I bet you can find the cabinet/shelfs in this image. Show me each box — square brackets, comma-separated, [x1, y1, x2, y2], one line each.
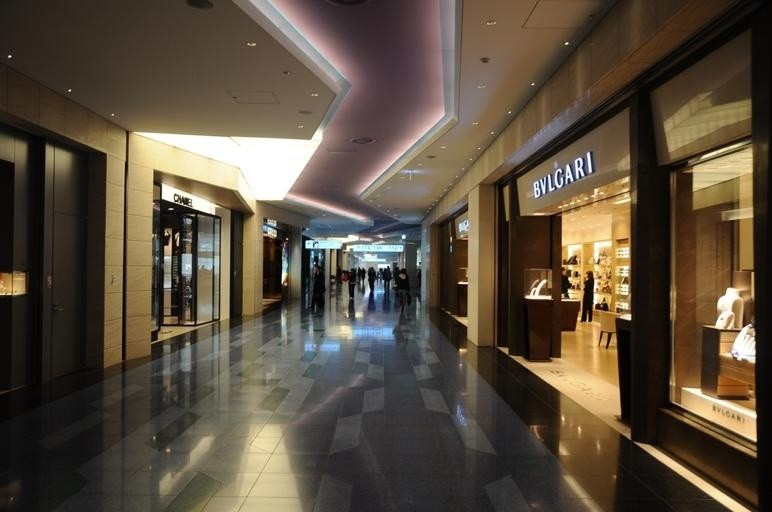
[457, 282, 468, 313]
[562, 243, 594, 317]
[700, 325, 754, 400]
[610, 238, 631, 315]
[561, 298, 581, 333]
[614, 316, 633, 429]
[582, 241, 611, 316]
[524, 294, 554, 363]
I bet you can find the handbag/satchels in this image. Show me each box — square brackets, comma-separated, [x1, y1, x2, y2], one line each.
[407, 294, 411, 306]
[596, 253, 611, 293]
[564, 255, 580, 289]
[595, 302, 609, 311]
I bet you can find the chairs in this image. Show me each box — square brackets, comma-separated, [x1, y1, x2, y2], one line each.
[597, 309, 621, 348]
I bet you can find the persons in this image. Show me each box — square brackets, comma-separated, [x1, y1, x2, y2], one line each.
[312, 256, 320, 285]
[580, 271, 595, 322]
[308, 264, 325, 312]
[561, 267, 574, 299]
[330, 262, 413, 314]
